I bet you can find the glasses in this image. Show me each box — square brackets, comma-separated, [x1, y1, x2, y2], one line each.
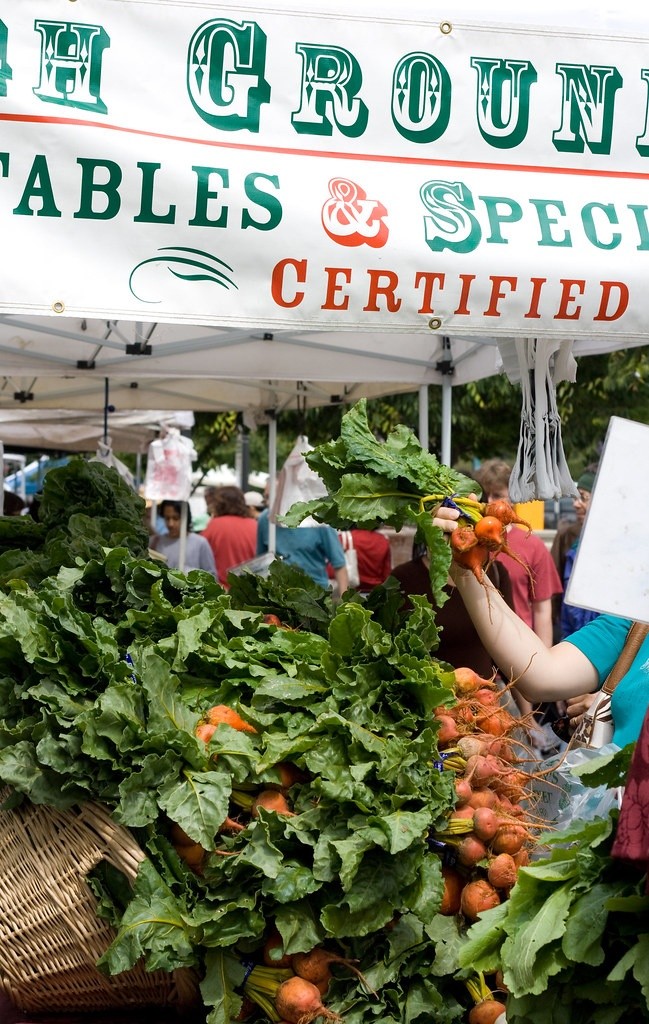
[571, 493, 592, 502]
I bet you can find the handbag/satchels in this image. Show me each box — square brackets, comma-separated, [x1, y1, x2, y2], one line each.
[569, 688, 615, 752]
[338, 530, 360, 587]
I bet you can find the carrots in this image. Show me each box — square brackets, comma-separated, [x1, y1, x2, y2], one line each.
[180, 665, 584, 1024]
[451, 500, 536, 625]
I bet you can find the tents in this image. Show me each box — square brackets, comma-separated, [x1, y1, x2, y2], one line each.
[1, 0, 648, 568]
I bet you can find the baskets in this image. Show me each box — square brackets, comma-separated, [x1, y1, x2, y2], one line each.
[0, 779, 204, 1017]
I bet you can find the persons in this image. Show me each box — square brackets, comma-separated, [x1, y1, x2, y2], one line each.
[198, 486, 267, 593]
[256, 474, 348, 599]
[362, 457, 649, 750]
[149, 499, 219, 578]
[325, 526, 393, 593]
